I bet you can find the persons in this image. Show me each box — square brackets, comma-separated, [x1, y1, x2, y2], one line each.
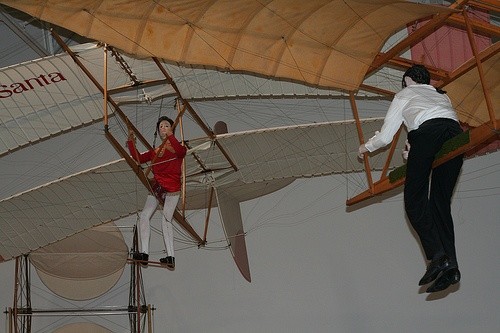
[357, 63, 465, 293]
[127, 116, 187, 269]
